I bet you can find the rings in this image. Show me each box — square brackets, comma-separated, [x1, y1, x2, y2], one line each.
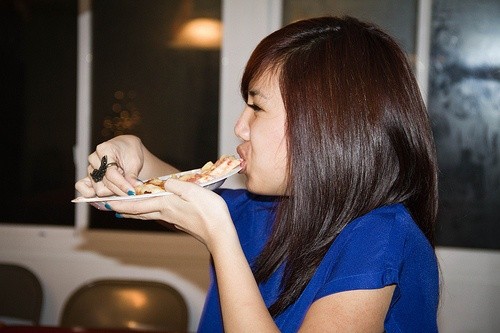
[90, 154, 119, 182]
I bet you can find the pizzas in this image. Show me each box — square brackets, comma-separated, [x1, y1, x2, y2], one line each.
[134, 155, 243, 195]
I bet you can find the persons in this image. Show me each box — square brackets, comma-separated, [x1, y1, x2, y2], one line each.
[74, 13, 441, 333]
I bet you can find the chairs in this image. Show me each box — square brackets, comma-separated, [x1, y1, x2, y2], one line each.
[58, 276, 190, 333]
[0, 261, 44, 326]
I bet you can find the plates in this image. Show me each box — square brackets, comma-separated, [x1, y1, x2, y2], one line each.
[71, 166, 243, 210]
